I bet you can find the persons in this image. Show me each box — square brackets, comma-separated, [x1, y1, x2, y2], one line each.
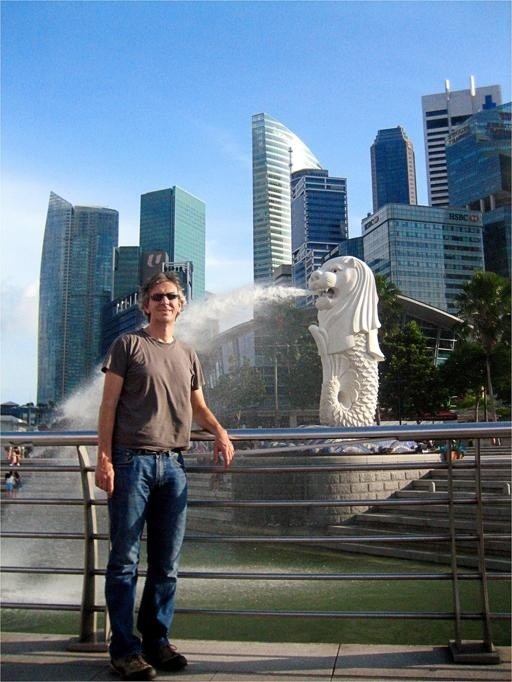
[6, 447, 22, 497]
[94, 273, 234, 680]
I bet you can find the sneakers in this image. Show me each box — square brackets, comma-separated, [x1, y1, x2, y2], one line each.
[143, 644, 188, 671]
[108, 654, 157, 681]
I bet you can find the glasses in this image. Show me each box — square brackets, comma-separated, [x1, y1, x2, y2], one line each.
[147, 292, 178, 302]
